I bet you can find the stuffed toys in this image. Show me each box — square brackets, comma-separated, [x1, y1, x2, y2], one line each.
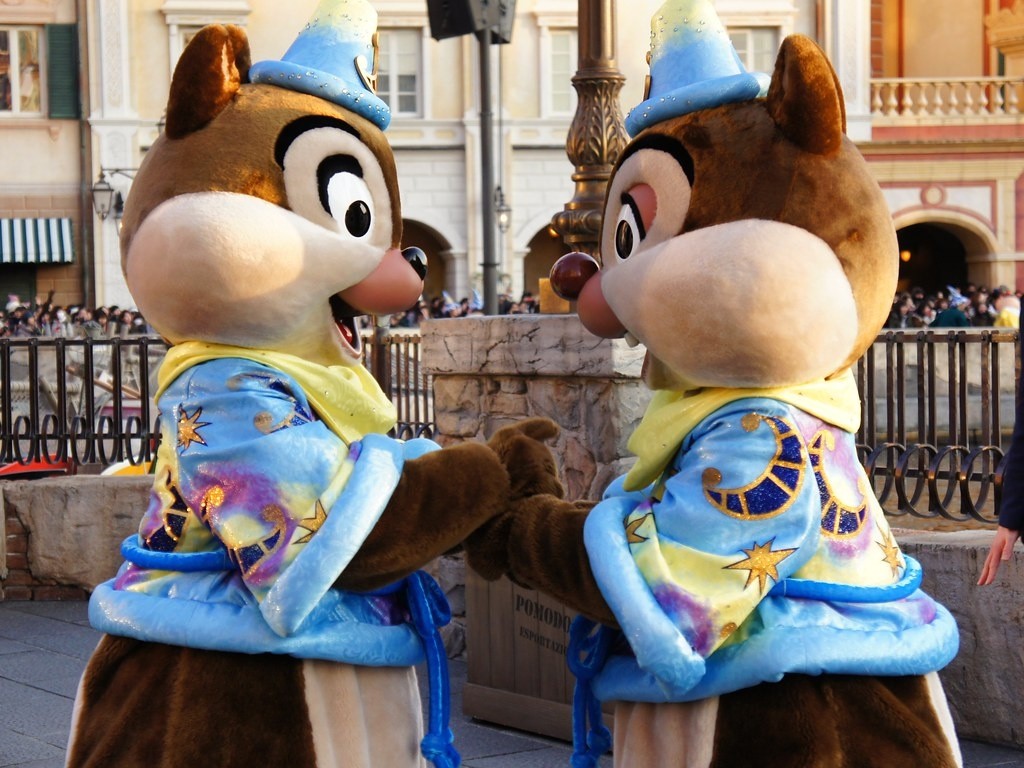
[64, 0, 564, 768]
[461, 0, 964, 768]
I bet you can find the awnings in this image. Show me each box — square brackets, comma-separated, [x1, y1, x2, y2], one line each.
[0, 217, 77, 264]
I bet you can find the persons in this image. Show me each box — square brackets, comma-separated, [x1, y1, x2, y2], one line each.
[977, 364, 1024, 586]
[0, 282, 156, 339]
[377, 289, 540, 330]
[883, 282, 1024, 329]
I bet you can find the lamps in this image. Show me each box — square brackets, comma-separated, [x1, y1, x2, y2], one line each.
[112, 192, 124, 235]
[90, 173, 115, 221]
